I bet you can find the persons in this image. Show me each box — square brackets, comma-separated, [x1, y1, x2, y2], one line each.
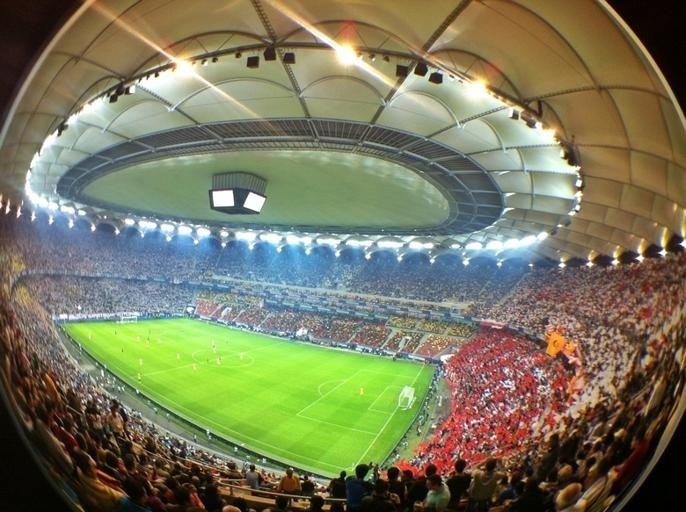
[2, 201, 686, 512]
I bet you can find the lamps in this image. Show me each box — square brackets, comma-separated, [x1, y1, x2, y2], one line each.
[107, 39, 444, 104]
